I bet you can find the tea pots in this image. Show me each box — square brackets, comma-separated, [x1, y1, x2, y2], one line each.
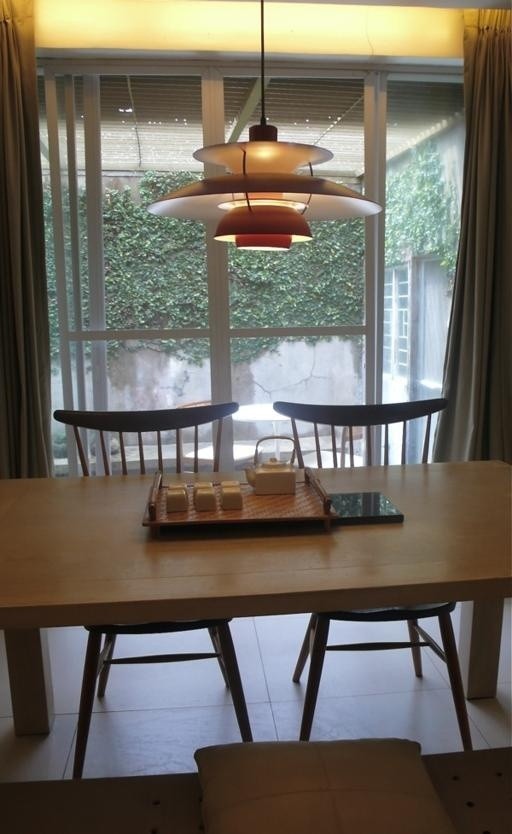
[245, 435, 301, 498]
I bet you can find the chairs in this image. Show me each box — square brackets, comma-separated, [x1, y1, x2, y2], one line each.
[271, 395, 474, 754]
[53, 402, 253, 781]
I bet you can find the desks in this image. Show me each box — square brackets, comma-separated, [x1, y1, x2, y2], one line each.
[0, 458, 512, 739]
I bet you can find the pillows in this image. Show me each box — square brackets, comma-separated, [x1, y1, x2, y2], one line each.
[193, 738, 457, 833]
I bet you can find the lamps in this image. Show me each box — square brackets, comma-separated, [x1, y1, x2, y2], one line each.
[148, 1, 384, 252]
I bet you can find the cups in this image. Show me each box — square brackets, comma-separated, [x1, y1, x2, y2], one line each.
[164, 480, 245, 513]
